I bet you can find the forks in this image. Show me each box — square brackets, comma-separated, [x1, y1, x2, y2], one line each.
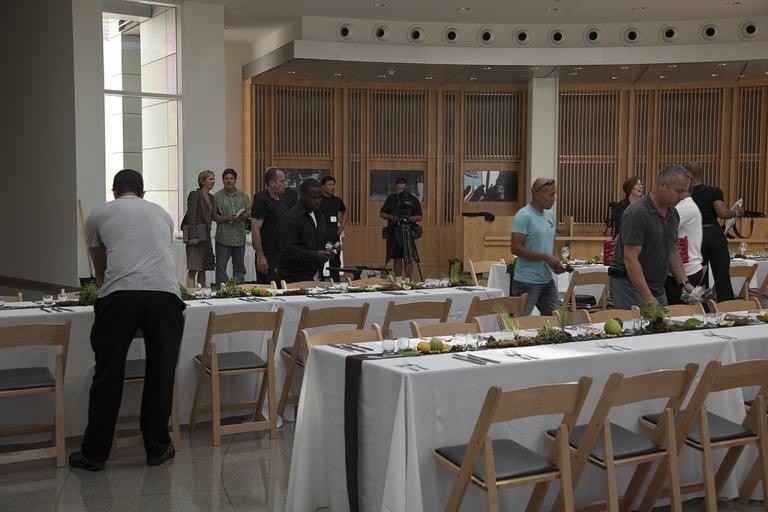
[503, 349, 539, 359]
[511, 350, 531, 360]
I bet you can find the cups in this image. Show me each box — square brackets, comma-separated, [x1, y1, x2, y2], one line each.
[382, 339, 394, 357]
[560, 247, 570, 259]
[396, 337, 409, 356]
[58, 294, 67, 302]
[739, 242, 748, 258]
[467, 333, 478, 351]
[43, 295, 53, 304]
[202, 288, 211, 298]
[194, 288, 202, 298]
[455, 333, 467, 351]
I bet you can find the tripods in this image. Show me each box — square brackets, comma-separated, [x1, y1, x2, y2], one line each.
[383, 223, 424, 282]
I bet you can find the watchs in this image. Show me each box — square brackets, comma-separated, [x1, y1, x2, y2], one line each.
[735, 210, 738, 217]
[679, 279, 690, 287]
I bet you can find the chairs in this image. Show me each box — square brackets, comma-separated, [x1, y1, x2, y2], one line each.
[705, 297, 762, 312]
[750, 274, 768, 302]
[546, 361, 702, 512]
[106, 311, 187, 451]
[640, 358, 768, 511]
[300, 323, 383, 350]
[579, 305, 641, 325]
[465, 291, 531, 324]
[382, 297, 452, 336]
[0, 317, 74, 469]
[497, 312, 560, 333]
[564, 270, 610, 322]
[653, 301, 705, 314]
[714, 263, 758, 298]
[189, 307, 285, 447]
[409, 319, 483, 337]
[432, 374, 593, 512]
[278, 302, 371, 433]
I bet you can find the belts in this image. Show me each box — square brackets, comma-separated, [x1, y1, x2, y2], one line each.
[702, 223, 715, 227]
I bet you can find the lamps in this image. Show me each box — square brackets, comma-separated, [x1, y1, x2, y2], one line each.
[543, 6, 559, 13]
[457, 5, 471, 12]
[728, 0, 742, 7]
[372, 1, 386, 9]
[630, 6, 646, 12]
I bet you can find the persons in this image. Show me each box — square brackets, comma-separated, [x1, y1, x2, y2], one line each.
[380, 178, 423, 282]
[683, 161, 745, 302]
[179, 170, 217, 287]
[677, 178, 703, 306]
[607, 164, 694, 309]
[250, 166, 288, 282]
[212, 167, 252, 284]
[69, 168, 187, 474]
[613, 175, 643, 221]
[320, 177, 349, 281]
[277, 177, 336, 282]
[511, 177, 566, 317]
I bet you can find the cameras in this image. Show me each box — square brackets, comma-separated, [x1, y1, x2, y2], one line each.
[396, 189, 412, 223]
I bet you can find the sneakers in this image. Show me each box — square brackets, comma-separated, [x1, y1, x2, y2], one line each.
[147, 441, 175, 466]
[69, 451, 103, 472]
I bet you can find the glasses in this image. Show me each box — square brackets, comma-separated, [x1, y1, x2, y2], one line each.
[536, 178, 555, 191]
